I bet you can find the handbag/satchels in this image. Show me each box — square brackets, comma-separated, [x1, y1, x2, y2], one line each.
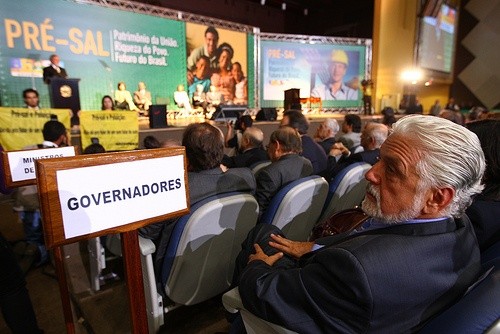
[315, 207, 368, 238]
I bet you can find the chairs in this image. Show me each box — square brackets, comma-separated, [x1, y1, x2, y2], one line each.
[165, 128, 499, 334]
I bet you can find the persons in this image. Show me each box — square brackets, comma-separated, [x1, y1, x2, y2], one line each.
[43, 55, 66, 84]
[133, 82, 152, 115]
[192, 84, 221, 114]
[83, 98, 485, 303]
[101, 95, 113, 110]
[465, 119, 500, 258]
[188, 26, 248, 106]
[223, 114, 487, 334]
[22, 89, 40, 109]
[174, 84, 191, 109]
[114, 81, 144, 113]
[311, 49, 358, 100]
[11, 120, 67, 211]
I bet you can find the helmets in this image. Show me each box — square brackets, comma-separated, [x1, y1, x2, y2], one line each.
[328, 48, 348, 68]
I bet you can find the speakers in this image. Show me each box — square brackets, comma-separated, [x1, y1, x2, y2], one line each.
[255, 107, 278, 121]
[150, 104, 168, 128]
